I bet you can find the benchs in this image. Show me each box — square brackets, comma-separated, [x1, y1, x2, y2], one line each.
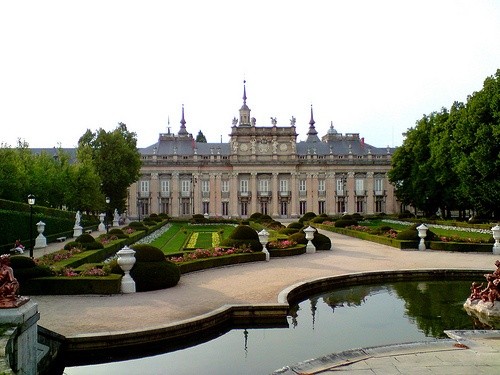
[85, 230, 92, 234]
[56, 236, 67, 242]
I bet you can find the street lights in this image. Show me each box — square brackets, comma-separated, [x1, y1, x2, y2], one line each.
[191, 175, 198, 214]
[341, 174, 347, 213]
[105, 197, 110, 233]
[27, 195, 37, 259]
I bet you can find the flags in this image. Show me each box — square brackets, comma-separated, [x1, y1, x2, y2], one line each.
[191, 138, 198, 150]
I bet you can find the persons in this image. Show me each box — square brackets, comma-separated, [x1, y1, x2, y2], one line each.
[14, 238, 25, 250]
[0, 254, 19, 300]
[470, 260, 500, 303]
